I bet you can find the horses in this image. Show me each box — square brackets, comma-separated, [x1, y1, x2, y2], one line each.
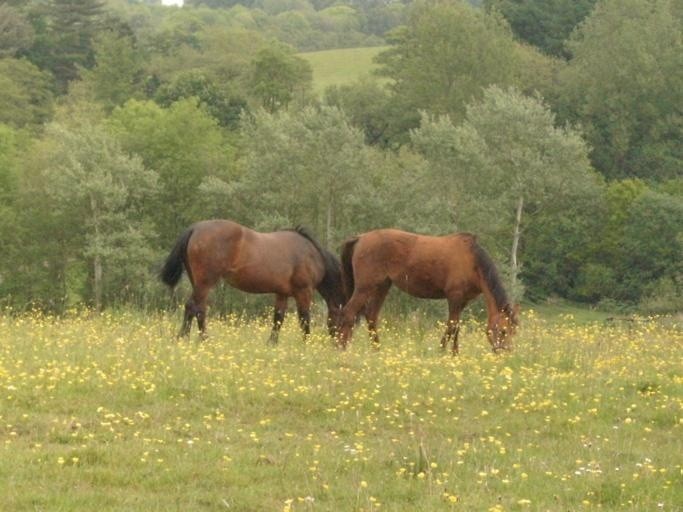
[337, 228, 519, 354]
[158, 219, 354, 348]
